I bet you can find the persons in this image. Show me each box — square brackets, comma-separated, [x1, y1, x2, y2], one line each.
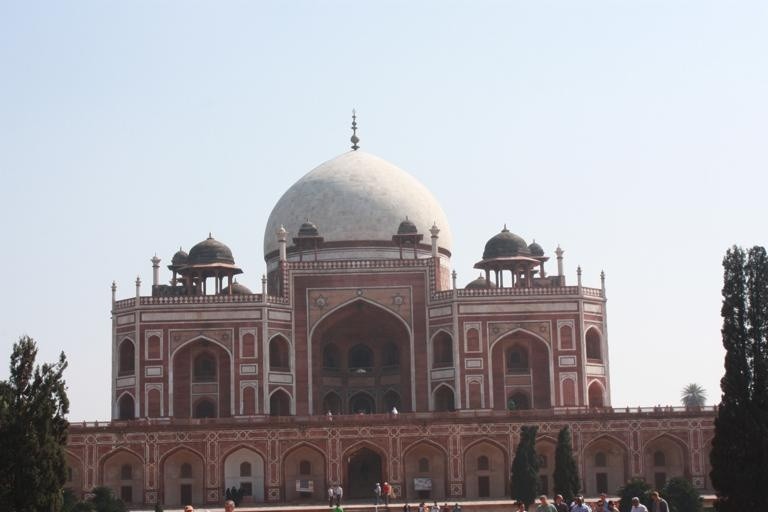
[184, 505, 193, 512]
[335, 484, 343, 504]
[383, 481, 390, 505]
[326, 409, 332, 421]
[328, 484, 334, 507]
[553, 494, 569, 512]
[630, 496, 648, 512]
[388, 482, 396, 503]
[515, 502, 528, 512]
[536, 494, 559, 512]
[225, 499, 235, 512]
[418, 500, 461, 512]
[570, 492, 620, 511]
[651, 491, 670, 511]
[404, 502, 411, 512]
[374, 482, 382, 505]
